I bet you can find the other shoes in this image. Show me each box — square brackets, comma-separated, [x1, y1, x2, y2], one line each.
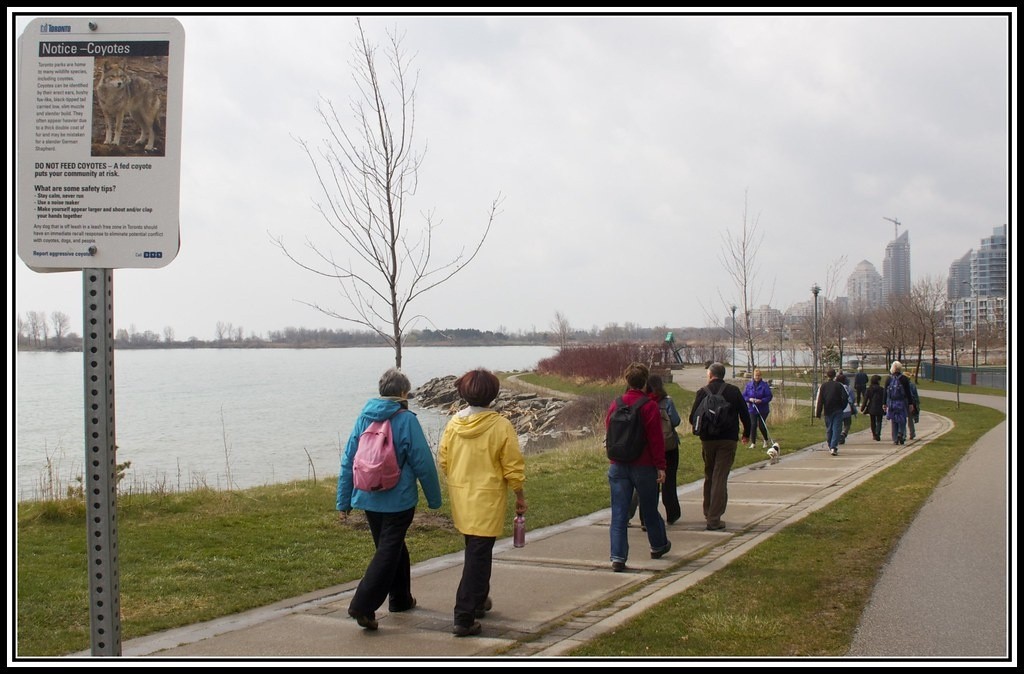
[839, 434, 844, 444]
[874, 434, 880, 441]
[708, 521, 725, 530]
[412, 597, 416, 608]
[348, 607, 378, 629]
[898, 436, 904, 444]
[831, 448, 838, 455]
[641, 521, 647, 530]
[910, 434, 915, 439]
[894, 442, 898, 445]
[762, 440, 767, 448]
[749, 443, 755, 448]
[627, 522, 633, 527]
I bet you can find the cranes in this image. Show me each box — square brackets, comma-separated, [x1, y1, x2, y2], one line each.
[882, 216, 901, 240]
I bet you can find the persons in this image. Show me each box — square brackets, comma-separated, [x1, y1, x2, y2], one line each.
[688, 361, 752, 533]
[599, 363, 671, 571]
[853, 367, 868, 408]
[638, 373, 680, 533]
[900, 371, 921, 441]
[742, 369, 774, 449]
[335, 368, 444, 630]
[815, 369, 848, 457]
[834, 371, 856, 446]
[860, 374, 886, 442]
[882, 360, 913, 445]
[437, 371, 527, 637]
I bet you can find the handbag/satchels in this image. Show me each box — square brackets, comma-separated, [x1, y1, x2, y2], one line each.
[863, 403, 870, 414]
[851, 404, 858, 415]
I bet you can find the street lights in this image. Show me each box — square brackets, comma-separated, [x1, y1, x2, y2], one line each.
[731, 304, 737, 378]
[811, 282, 822, 401]
[963, 281, 979, 368]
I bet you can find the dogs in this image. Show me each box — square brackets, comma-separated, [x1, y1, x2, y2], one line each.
[766, 442, 780, 461]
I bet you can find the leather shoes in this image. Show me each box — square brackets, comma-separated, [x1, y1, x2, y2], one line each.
[475, 597, 492, 619]
[651, 540, 671, 559]
[451, 621, 481, 635]
[612, 562, 625, 572]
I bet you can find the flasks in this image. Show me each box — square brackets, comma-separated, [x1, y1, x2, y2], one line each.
[514, 514, 525, 548]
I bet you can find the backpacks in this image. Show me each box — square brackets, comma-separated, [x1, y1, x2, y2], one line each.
[659, 398, 678, 453]
[604, 394, 652, 463]
[351, 408, 409, 491]
[691, 382, 732, 437]
[888, 374, 906, 400]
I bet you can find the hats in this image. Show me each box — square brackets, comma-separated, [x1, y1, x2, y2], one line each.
[827, 369, 836, 378]
